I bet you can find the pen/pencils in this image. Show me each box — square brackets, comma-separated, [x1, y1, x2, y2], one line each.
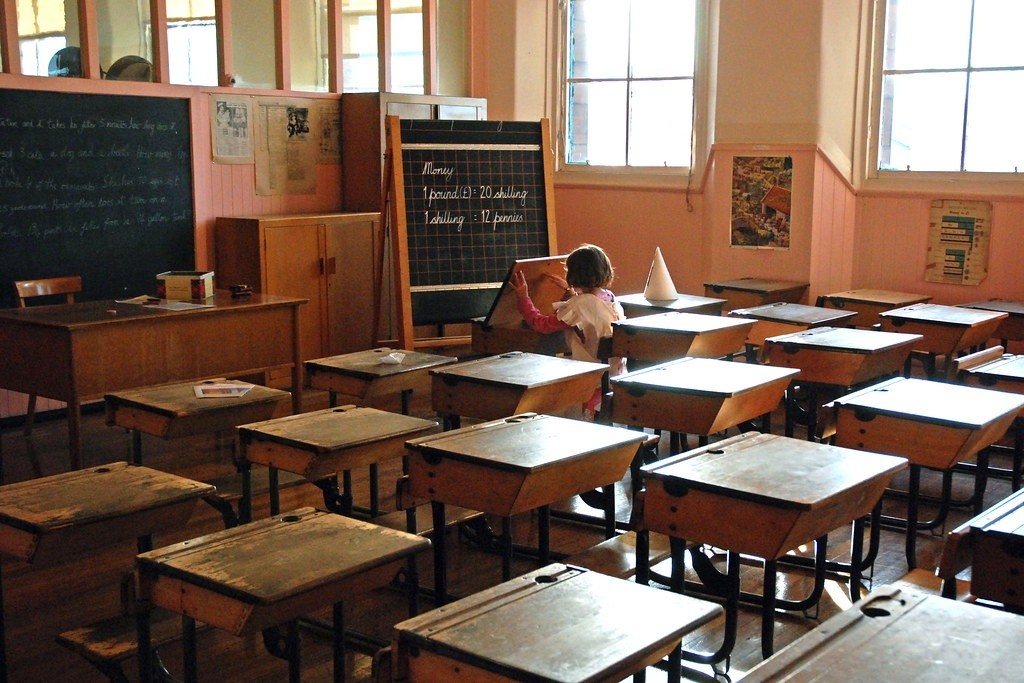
[142, 298, 161, 305]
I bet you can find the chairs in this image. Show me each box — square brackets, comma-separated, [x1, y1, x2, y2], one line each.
[901, 488, 1024, 602]
[587, 393, 660, 463]
[946, 344, 1004, 384]
[55, 531, 217, 683]
[15, 275, 86, 436]
[592, 337, 612, 414]
[363, 475, 488, 588]
[563, 431, 758, 578]
[814, 376, 906, 446]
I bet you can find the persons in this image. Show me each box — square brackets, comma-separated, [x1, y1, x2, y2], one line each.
[507, 245, 629, 423]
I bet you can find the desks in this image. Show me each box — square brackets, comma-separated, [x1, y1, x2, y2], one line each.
[0, 255, 1024, 683]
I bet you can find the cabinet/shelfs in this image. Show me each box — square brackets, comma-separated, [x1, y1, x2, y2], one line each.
[340, 92, 488, 346]
[216, 211, 390, 390]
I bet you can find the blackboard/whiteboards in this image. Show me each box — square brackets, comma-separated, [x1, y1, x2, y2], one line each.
[0, 73, 213, 311]
[387, 114, 559, 294]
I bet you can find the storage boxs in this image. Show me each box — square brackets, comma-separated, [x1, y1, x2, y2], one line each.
[155, 270, 216, 302]
